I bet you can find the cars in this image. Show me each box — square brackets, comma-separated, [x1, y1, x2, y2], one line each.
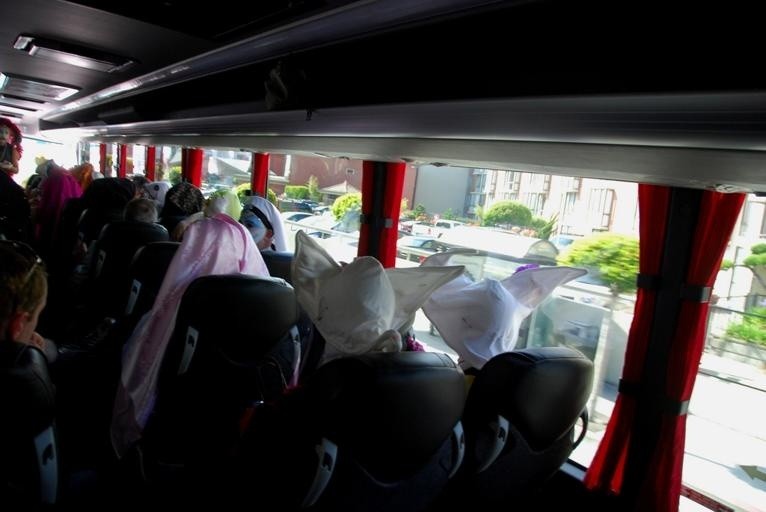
[201, 165, 580, 270]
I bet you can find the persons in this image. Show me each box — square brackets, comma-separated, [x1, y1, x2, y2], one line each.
[0, 117, 546, 512]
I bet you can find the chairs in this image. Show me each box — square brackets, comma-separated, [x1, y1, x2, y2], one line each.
[0, 177, 594, 512]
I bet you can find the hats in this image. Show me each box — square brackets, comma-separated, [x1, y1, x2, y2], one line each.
[417, 246, 587, 371]
[245, 195, 286, 252]
[289, 229, 465, 357]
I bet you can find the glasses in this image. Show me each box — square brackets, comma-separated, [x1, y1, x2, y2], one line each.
[1, 231, 42, 286]
